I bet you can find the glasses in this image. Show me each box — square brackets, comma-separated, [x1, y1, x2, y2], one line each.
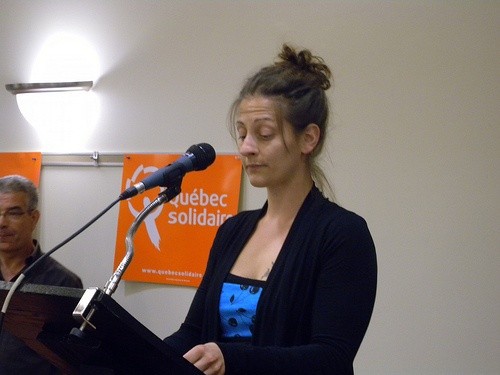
[0, 208, 35, 219]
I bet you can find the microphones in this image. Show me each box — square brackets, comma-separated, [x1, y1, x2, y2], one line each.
[119, 143, 216, 200]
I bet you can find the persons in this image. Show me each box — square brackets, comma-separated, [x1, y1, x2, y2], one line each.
[164, 44, 378, 375]
[0, 175, 83, 375]
